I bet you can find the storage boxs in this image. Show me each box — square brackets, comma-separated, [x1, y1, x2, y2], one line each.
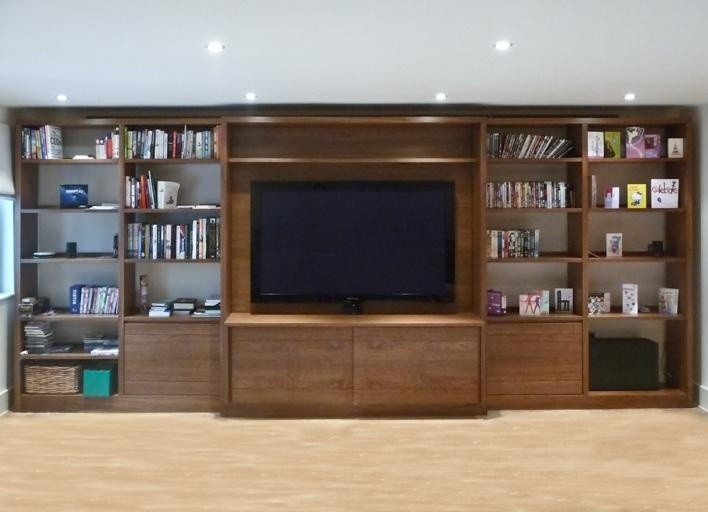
[83, 364, 117, 398]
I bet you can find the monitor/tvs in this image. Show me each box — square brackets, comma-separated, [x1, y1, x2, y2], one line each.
[248, 178, 456, 316]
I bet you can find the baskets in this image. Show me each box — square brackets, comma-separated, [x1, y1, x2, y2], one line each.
[22, 363, 83, 393]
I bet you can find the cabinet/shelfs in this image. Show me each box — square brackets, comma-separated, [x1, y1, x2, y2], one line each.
[224, 313, 486, 420]
[481, 118, 693, 410]
[15, 118, 227, 414]
[222, 117, 484, 325]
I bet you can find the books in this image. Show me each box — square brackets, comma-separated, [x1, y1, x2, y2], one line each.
[589, 293, 611, 313]
[95, 128, 119, 159]
[626, 127, 661, 158]
[519, 290, 550, 317]
[590, 175, 597, 207]
[605, 233, 623, 257]
[555, 288, 574, 314]
[601, 186, 620, 210]
[80, 327, 119, 356]
[589, 132, 603, 157]
[172, 296, 198, 317]
[651, 179, 679, 208]
[149, 303, 173, 318]
[22, 322, 74, 358]
[124, 124, 214, 159]
[487, 229, 539, 257]
[668, 138, 684, 158]
[605, 131, 621, 158]
[486, 180, 577, 209]
[622, 283, 639, 315]
[126, 172, 180, 208]
[657, 288, 679, 314]
[487, 290, 508, 316]
[204, 299, 221, 315]
[68, 285, 118, 314]
[626, 184, 647, 208]
[21, 126, 63, 159]
[488, 133, 575, 159]
[127, 217, 220, 259]
